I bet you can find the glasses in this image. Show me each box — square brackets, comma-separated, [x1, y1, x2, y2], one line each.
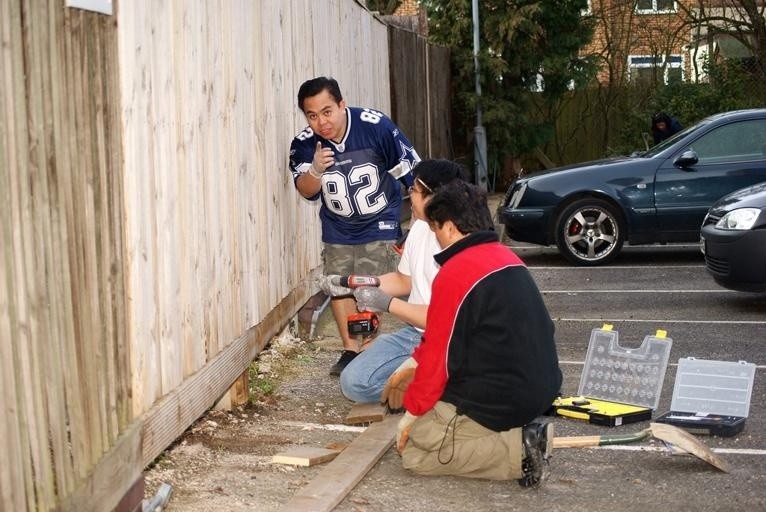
[408, 186, 422, 194]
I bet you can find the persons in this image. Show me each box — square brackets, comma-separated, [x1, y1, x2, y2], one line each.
[288, 78, 423, 378]
[378, 179, 565, 490]
[652, 113, 684, 145]
[316, 156, 471, 402]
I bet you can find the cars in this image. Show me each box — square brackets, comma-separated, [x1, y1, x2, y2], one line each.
[699, 179, 765, 294]
[497, 108, 765, 268]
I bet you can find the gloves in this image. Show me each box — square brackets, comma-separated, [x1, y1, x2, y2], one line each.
[353, 286, 394, 313]
[319, 275, 354, 297]
[309, 141, 335, 179]
[397, 411, 418, 456]
[381, 357, 419, 409]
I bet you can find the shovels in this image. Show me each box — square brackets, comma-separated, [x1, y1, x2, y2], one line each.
[553, 422, 730, 474]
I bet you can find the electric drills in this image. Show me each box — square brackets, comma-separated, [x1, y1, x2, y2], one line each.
[331, 275, 380, 335]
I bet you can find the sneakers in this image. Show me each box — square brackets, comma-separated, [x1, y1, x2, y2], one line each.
[330, 350, 357, 376]
[519, 422, 554, 488]
